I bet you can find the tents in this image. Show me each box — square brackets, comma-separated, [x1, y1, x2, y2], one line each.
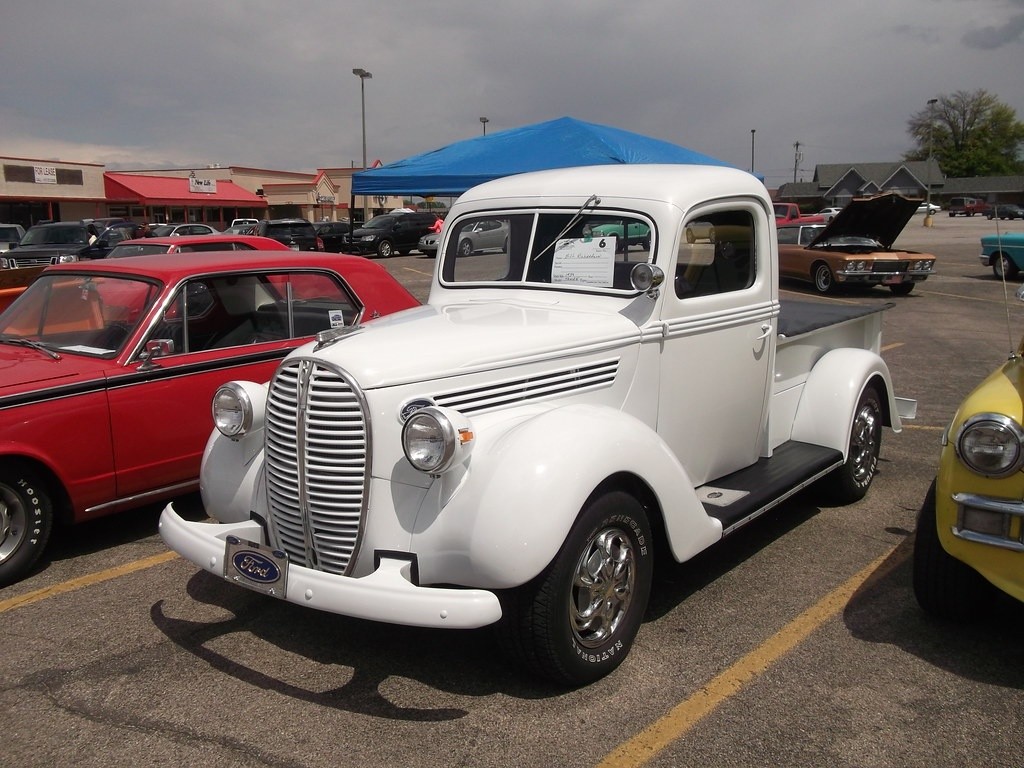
[349, 116, 764, 256]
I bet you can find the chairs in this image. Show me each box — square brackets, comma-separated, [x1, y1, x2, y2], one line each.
[159, 322, 186, 348]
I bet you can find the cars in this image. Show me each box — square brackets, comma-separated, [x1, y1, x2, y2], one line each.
[915, 197, 1024, 281]
[93, 235, 343, 322]
[148, 219, 259, 238]
[912, 336, 1024, 633]
[777, 192, 937, 295]
[417, 220, 509, 258]
[686, 222, 716, 244]
[583, 220, 651, 255]
[0, 224, 26, 254]
[0, 250, 424, 585]
[342, 212, 439, 258]
[312, 222, 357, 253]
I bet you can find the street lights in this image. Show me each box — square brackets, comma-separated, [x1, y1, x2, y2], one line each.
[927, 99, 939, 218]
[352, 69, 373, 224]
[480, 117, 489, 135]
[750, 129, 755, 172]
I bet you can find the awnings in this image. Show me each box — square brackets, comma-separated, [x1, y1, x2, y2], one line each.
[103, 173, 268, 209]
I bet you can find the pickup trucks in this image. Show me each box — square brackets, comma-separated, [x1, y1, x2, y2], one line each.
[158, 164, 920, 688]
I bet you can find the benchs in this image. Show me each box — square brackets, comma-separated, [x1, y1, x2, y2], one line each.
[615, 261, 747, 295]
[206, 311, 337, 349]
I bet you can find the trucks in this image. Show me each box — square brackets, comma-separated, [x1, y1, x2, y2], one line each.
[773, 203, 825, 227]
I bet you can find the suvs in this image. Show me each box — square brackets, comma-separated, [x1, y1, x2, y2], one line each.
[0, 217, 158, 270]
[246, 218, 319, 252]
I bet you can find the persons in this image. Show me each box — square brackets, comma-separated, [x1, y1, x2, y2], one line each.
[427, 215, 444, 233]
[88, 224, 98, 246]
[136, 222, 151, 239]
